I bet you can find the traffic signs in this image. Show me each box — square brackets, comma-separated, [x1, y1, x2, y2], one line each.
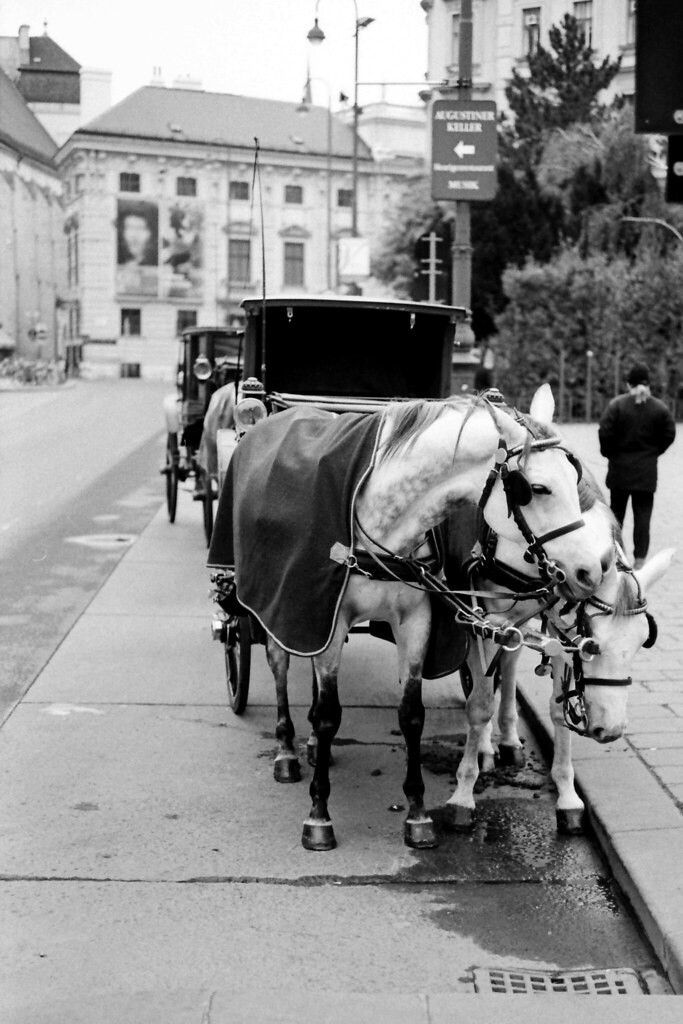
[430, 100, 501, 202]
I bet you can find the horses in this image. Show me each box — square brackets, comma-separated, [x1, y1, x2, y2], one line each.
[438, 450, 650, 836]
[231, 381, 604, 852]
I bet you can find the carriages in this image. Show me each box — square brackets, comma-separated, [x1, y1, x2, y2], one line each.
[201, 298, 682, 853]
[157, 325, 248, 548]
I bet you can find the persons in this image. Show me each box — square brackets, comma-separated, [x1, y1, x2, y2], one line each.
[597, 362, 676, 573]
[118, 212, 157, 266]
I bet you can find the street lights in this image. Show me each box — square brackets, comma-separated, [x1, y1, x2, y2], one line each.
[296, 73, 333, 291]
[307, 0, 378, 295]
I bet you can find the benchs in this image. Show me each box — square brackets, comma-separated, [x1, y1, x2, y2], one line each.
[239, 296, 468, 416]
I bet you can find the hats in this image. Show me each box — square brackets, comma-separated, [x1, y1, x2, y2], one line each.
[627, 361, 650, 384]
